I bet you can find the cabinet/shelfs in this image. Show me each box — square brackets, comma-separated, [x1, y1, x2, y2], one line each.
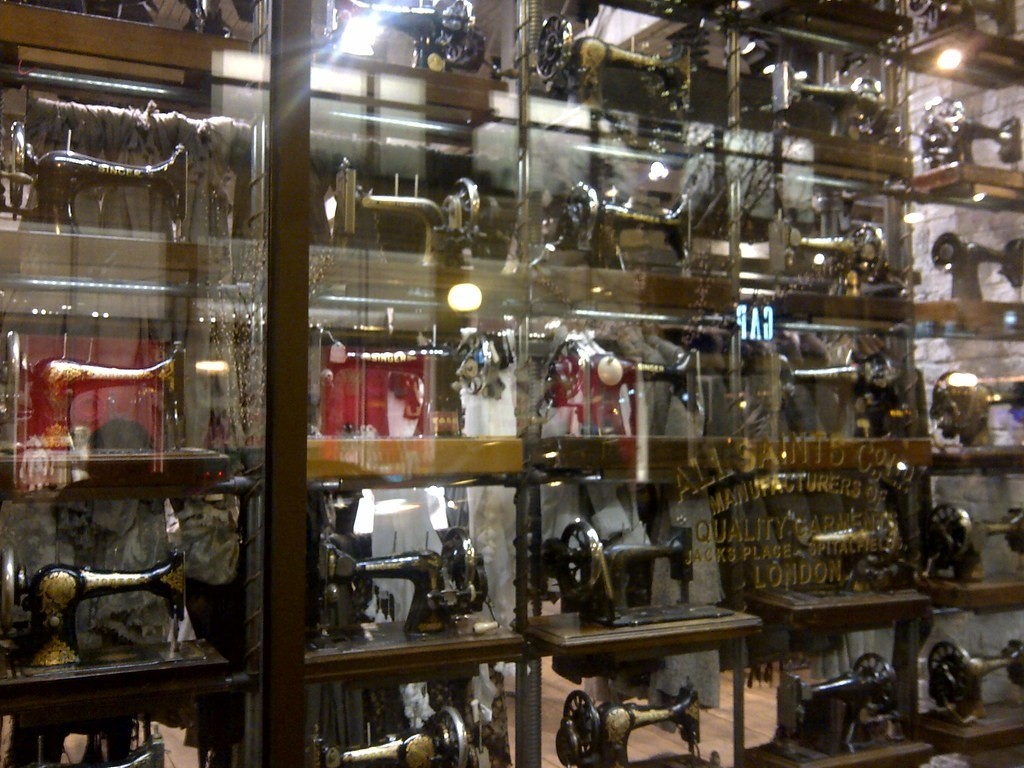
[0, 0, 1024, 768]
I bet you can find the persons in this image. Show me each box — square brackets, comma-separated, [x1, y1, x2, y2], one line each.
[29, 419, 187, 768]
